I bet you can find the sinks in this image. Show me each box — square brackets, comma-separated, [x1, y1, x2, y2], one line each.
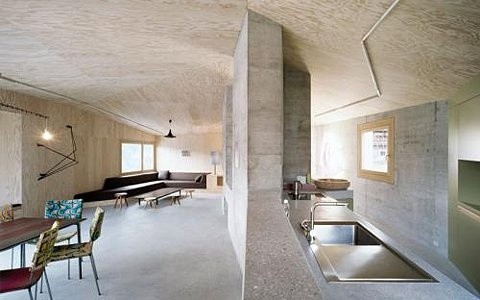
[301, 220, 383, 247]
[287, 190, 326, 201]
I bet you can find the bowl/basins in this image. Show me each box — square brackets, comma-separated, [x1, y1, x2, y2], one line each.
[314, 178, 350, 190]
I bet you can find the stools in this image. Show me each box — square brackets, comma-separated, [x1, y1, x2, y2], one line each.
[114, 193, 128, 209]
[167, 195, 181, 206]
[184, 189, 194, 198]
[144, 197, 157, 208]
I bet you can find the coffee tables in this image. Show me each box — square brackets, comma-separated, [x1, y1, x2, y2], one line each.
[134, 188, 183, 205]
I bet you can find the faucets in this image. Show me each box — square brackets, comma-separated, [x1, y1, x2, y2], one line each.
[293, 180, 302, 195]
[308, 202, 350, 231]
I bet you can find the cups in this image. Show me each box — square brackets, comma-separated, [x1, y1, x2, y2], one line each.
[293, 181, 302, 192]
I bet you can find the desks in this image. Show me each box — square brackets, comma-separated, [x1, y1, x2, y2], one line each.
[0, 217, 88, 279]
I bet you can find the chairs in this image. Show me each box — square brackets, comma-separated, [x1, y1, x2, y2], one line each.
[0, 199, 104, 300]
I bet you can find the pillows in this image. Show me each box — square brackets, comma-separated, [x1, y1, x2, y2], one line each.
[158, 170, 169, 180]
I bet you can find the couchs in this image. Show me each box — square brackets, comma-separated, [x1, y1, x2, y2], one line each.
[74, 172, 212, 203]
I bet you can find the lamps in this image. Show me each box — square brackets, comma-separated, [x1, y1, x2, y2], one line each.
[164, 129, 176, 139]
